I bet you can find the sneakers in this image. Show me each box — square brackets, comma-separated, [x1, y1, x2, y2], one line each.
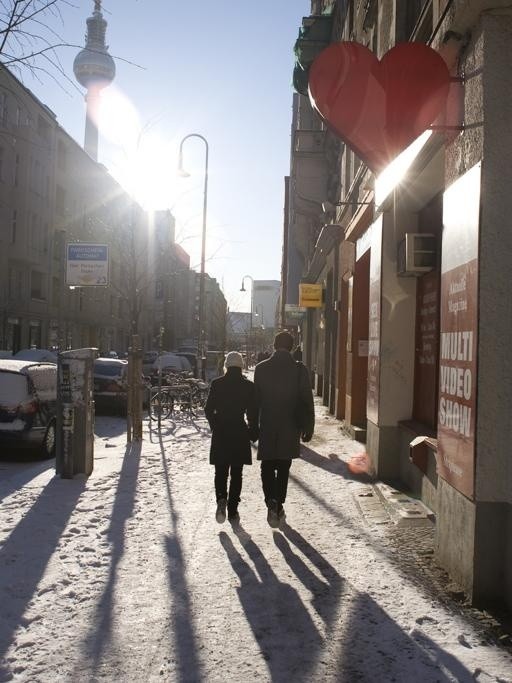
[228, 511, 238, 520]
[267, 499, 286, 528]
[216, 500, 226, 523]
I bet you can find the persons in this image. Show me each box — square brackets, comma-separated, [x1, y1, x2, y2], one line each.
[203, 349, 261, 524]
[253, 330, 316, 528]
[292, 344, 303, 362]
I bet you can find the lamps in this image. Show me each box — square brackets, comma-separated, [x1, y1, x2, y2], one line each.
[322, 199, 375, 213]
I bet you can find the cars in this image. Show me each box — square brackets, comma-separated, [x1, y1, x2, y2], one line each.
[0, 348, 200, 459]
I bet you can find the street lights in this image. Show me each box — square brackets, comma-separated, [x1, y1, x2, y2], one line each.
[174, 133, 208, 381]
[240, 274, 263, 370]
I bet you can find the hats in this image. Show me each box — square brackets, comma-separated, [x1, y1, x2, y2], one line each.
[226, 352, 242, 368]
[274, 333, 294, 351]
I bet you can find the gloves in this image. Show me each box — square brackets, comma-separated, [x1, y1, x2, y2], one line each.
[249, 425, 259, 443]
[301, 432, 312, 442]
